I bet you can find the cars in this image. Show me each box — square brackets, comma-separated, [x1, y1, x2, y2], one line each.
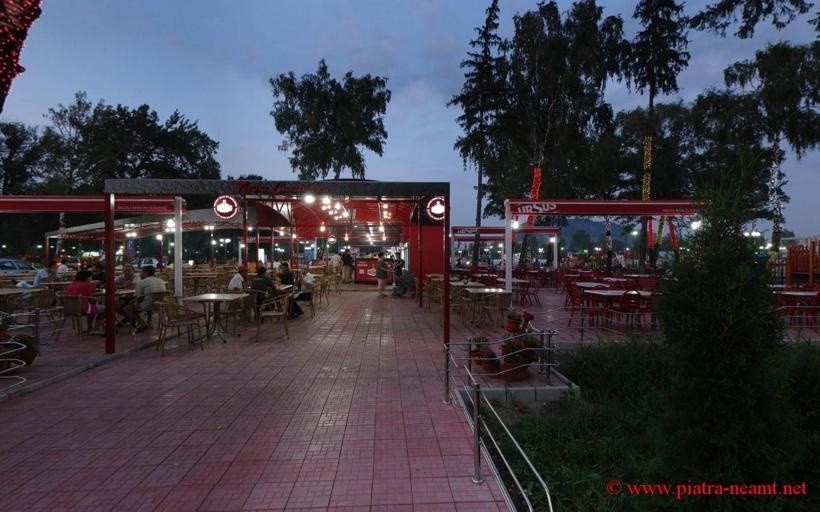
[0, 258, 39, 276]
[115, 257, 159, 269]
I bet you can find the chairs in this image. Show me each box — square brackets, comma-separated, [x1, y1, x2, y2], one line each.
[1, 224, 819, 379]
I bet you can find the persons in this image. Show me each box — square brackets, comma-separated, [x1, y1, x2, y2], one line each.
[391, 252, 405, 290]
[228, 257, 315, 320]
[533, 259, 540, 269]
[376, 252, 390, 298]
[33, 258, 107, 335]
[454, 259, 461, 268]
[322, 249, 378, 284]
[122, 265, 166, 334]
[115, 263, 141, 328]
